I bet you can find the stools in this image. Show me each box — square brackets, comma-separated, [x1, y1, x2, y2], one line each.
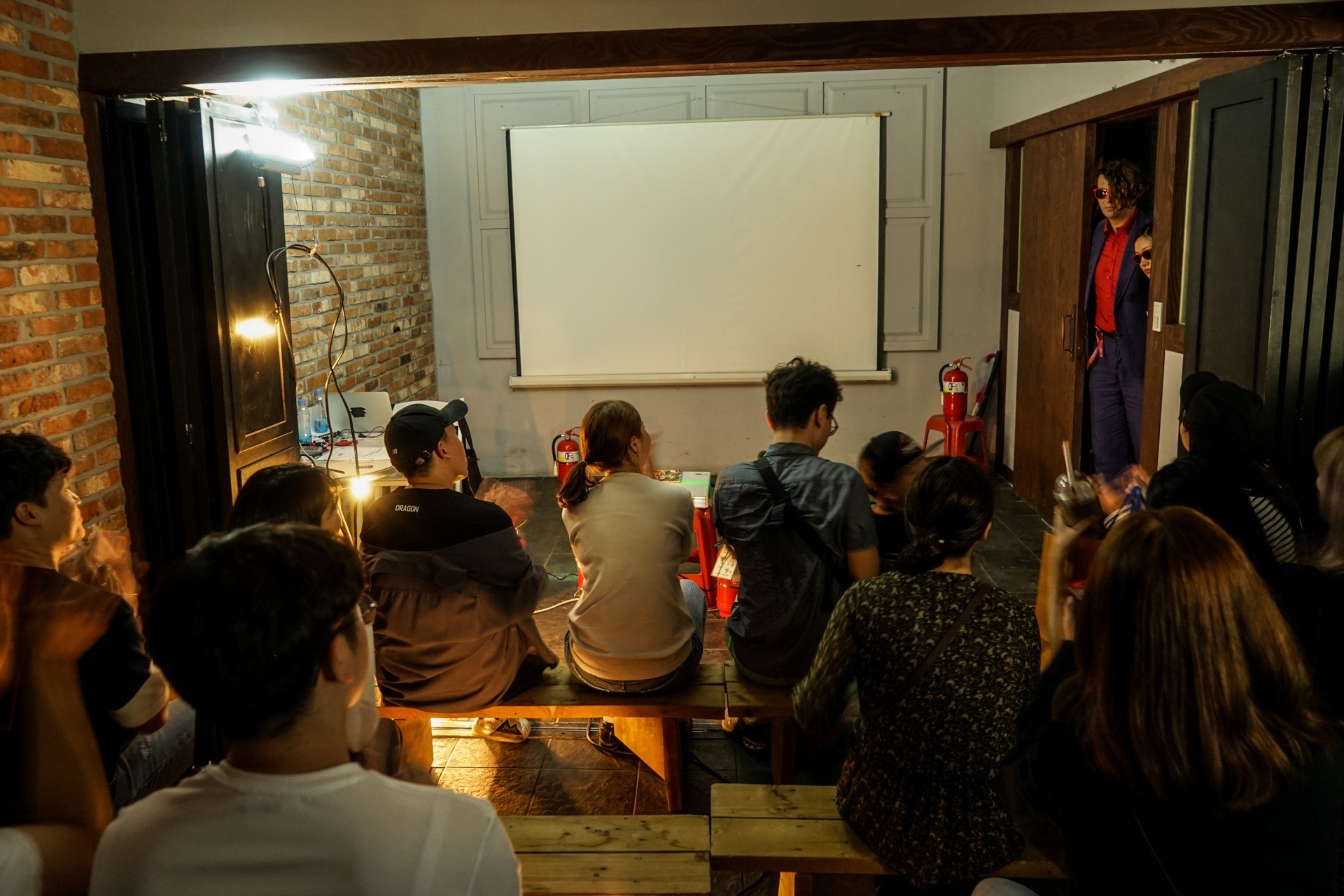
[920, 414, 988, 478]
[656, 497, 728, 617]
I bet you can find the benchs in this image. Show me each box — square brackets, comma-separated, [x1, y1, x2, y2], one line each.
[497, 808, 709, 896]
[710, 641, 836, 781]
[708, 778, 888, 896]
[363, 653, 725, 810]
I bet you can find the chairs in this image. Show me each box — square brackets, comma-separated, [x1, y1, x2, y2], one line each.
[374, 398, 462, 510]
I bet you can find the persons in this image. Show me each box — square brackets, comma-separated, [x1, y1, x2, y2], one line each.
[360, 400, 560, 714]
[859, 429, 944, 578]
[1083, 158, 1152, 482]
[793, 454, 1045, 890]
[1, 433, 527, 896]
[711, 356, 878, 691]
[1043, 373, 1344, 896]
[552, 400, 707, 698]
[1132, 222, 1153, 279]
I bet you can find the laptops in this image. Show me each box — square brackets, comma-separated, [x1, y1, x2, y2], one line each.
[321, 392, 392, 433]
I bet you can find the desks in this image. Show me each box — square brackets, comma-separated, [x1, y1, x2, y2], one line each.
[305, 425, 432, 566]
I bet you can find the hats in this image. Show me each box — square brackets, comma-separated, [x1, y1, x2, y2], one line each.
[384, 399, 469, 473]
[860, 431, 946, 493]
[1178, 370, 1250, 452]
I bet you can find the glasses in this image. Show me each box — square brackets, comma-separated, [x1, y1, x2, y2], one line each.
[325, 492, 341, 522]
[320, 593, 377, 642]
[828, 414, 838, 437]
[1093, 188, 1110, 199]
[1131, 248, 1152, 265]
[1179, 407, 1186, 422]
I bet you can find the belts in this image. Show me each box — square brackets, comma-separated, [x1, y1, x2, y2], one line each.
[1098, 330, 1117, 340]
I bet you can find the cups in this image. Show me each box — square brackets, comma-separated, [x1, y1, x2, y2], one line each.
[1053, 469, 1102, 526]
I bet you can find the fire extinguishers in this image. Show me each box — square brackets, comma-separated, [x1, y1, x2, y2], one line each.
[943, 354, 975, 420]
[557, 426, 581, 485]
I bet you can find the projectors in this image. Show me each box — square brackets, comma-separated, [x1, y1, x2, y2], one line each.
[682, 472, 712, 509]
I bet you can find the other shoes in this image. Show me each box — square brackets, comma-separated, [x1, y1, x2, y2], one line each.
[598, 716, 620, 752]
[721, 712, 773, 751]
[471, 717, 531, 743]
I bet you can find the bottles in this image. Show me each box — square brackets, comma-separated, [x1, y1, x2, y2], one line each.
[297, 399, 312, 444]
[313, 389, 328, 433]
